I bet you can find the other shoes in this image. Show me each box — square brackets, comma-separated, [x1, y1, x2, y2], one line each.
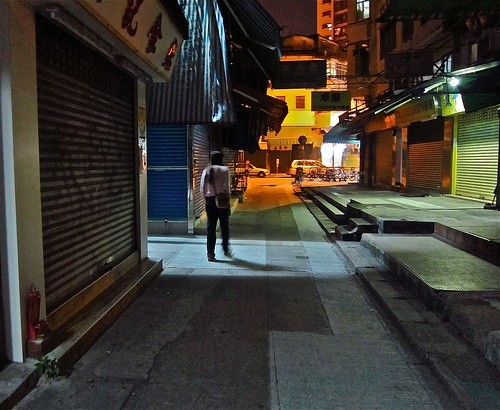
[208, 256, 214, 261]
[224, 251, 229, 255]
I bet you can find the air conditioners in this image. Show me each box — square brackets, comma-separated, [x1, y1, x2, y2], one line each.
[477, 32, 500, 59]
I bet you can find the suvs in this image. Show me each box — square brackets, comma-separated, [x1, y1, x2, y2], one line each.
[286, 159, 329, 178]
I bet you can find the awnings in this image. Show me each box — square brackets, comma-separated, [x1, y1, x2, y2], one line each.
[264, 127, 321, 144]
[325, 61, 498, 143]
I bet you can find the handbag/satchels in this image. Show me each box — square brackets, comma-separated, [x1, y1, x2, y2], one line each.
[216, 194, 229, 208]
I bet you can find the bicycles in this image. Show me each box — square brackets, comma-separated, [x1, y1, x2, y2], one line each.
[293, 167, 360, 182]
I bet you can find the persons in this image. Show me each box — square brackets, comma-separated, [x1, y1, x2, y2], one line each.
[201, 151, 232, 261]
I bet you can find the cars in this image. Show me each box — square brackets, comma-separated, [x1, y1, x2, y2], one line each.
[235, 162, 271, 178]
